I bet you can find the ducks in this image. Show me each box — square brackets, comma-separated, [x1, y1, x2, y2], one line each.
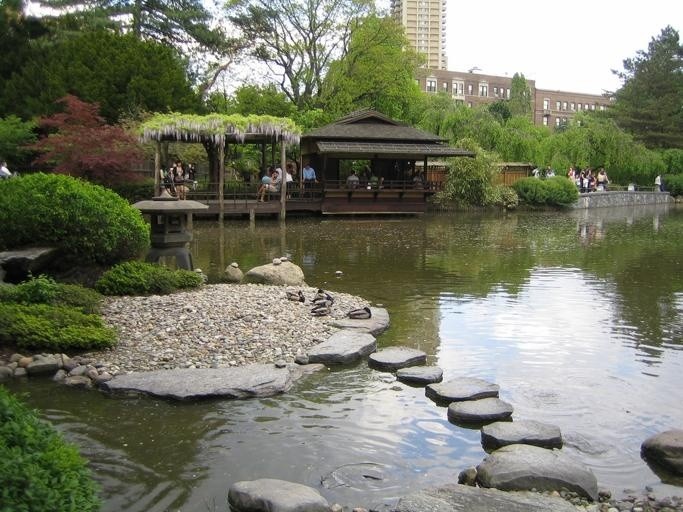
[286, 289, 305, 302]
[310, 289, 335, 316]
[347, 307, 372, 318]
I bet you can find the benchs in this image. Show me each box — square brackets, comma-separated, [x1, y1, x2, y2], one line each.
[323, 179, 433, 199]
[153, 180, 280, 202]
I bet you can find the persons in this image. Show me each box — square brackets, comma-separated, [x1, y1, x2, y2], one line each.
[160, 159, 426, 203]
[532, 165, 608, 193]
[655, 174, 664, 191]
[0, 161, 20, 180]
